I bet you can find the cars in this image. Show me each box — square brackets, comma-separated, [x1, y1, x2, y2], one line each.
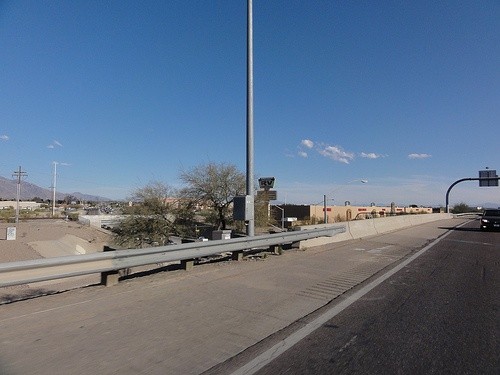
[480, 208, 500, 231]
